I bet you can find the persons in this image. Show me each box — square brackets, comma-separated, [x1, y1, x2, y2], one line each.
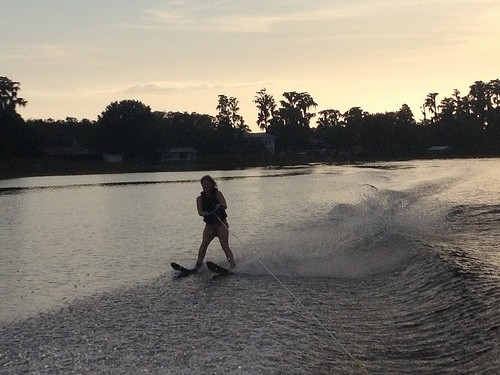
[195, 174, 236, 268]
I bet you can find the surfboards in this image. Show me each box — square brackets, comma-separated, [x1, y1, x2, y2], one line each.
[170, 260, 234, 276]
